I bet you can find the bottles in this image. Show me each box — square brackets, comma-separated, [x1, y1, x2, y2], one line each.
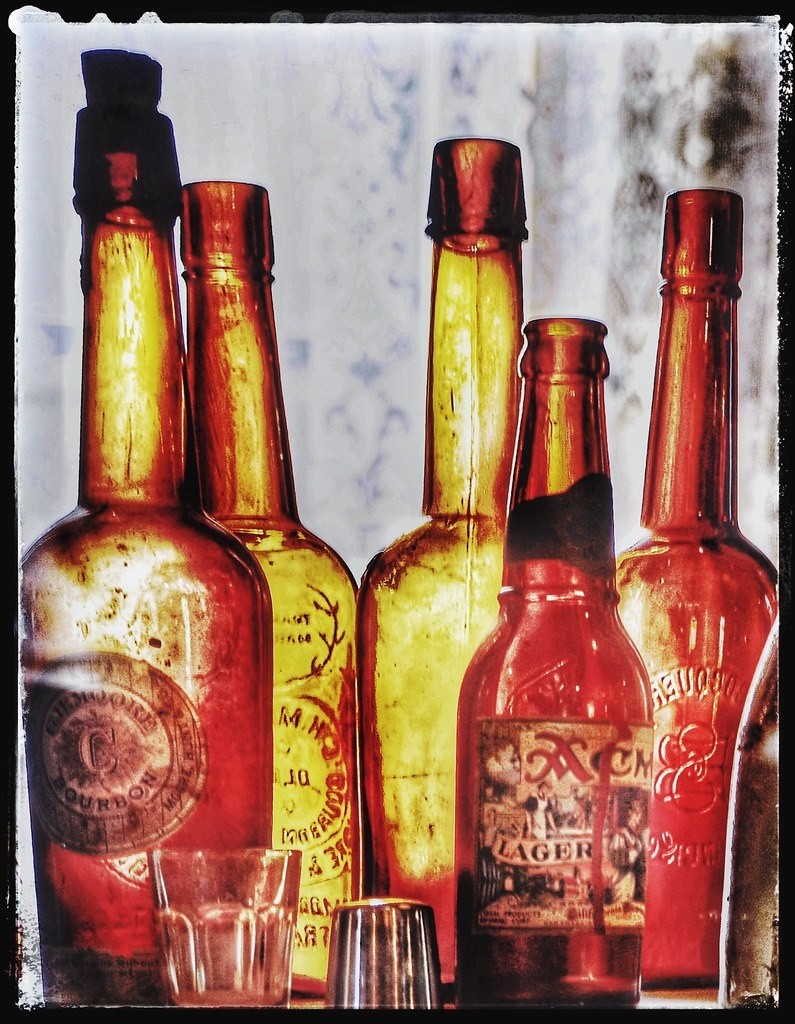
[454, 318, 653, 1010]
[717, 613, 779, 1009]
[616, 185, 778, 991]
[179, 183, 364, 998]
[359, 140, 528, 1001]
[21, 50, 272, 1005]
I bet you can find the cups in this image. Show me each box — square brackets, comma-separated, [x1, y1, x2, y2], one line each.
[146, 847, 303, 1007]
[323, 899, 442, 1011]
